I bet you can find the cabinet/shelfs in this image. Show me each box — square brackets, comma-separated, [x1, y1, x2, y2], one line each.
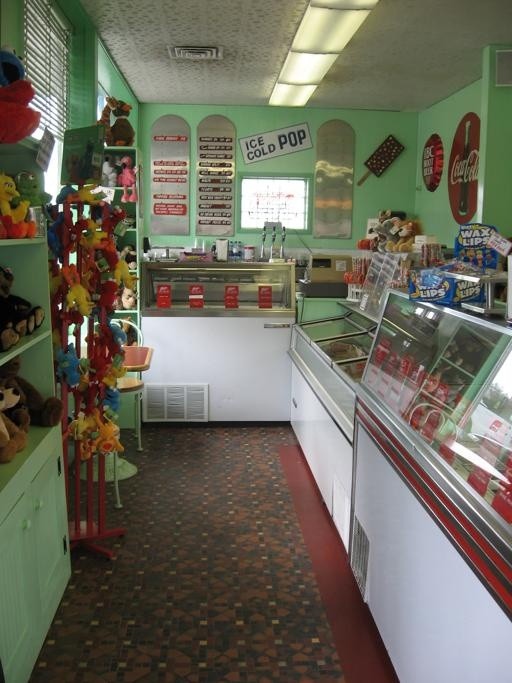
[140, 260, 296, 429]
[286, 290, 512, 681]
[0, 185, 75, 682]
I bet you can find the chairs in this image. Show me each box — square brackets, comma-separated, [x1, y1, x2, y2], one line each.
[87, 320, 143, 451]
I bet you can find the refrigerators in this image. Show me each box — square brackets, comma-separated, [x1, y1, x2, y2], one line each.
[286, 310, 380, 556]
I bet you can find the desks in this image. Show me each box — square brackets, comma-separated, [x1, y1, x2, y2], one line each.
[72, 344, 151, 479]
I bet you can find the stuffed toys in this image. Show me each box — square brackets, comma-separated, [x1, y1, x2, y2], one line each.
[0, 41, 142, 490]
[358, 211, 422, 254]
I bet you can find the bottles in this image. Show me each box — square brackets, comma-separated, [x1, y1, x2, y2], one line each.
[457, 121, 472, 216]
